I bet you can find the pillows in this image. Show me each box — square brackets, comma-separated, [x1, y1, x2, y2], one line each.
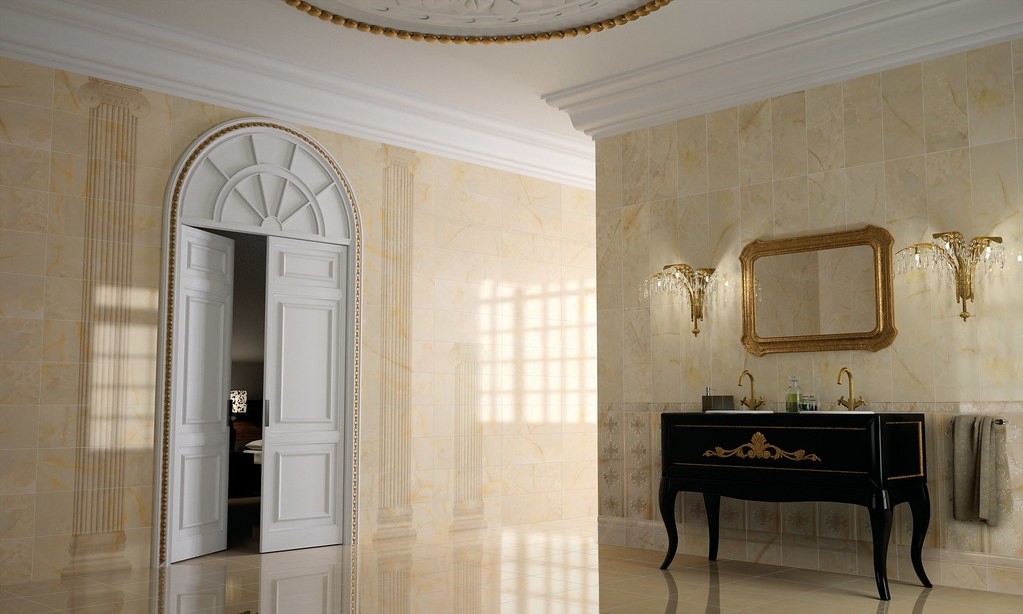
[245, 439, 262, 450]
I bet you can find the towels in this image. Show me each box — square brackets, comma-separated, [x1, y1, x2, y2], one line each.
[952, 414, 998, 527]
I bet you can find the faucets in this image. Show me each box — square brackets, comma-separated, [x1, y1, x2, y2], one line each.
[837, 367, 867, 411]
[738, 370, 767, 411]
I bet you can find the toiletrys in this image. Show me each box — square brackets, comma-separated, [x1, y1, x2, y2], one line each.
[705, 387, 717, 396]
[784, 375, 818, 413]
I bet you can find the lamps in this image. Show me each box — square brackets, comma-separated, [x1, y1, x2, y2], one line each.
[638, 264, 740, 337]
[892, 232, 1023, 322]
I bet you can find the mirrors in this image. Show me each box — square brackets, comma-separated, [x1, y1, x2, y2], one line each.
[739, 225, 898, 357]
